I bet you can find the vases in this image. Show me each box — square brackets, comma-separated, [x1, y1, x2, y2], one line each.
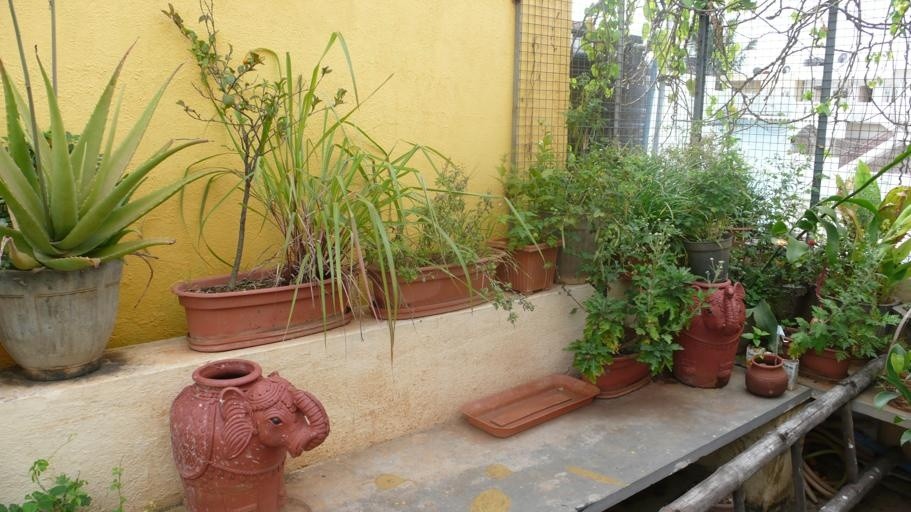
[171, 358, 330, 512]
[672, 273, 748, 391]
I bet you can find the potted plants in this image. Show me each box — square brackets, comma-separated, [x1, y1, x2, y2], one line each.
[482, 152, 562, 296]
[573, 257, 717, 400]
[0, 0, 228, 379]
[792, 153, 911, 382]
[160, 0, 540, 371]
[557, 127, 754, 284]
[363, 159, 535, 328]
[740, 326, 801, 400]
